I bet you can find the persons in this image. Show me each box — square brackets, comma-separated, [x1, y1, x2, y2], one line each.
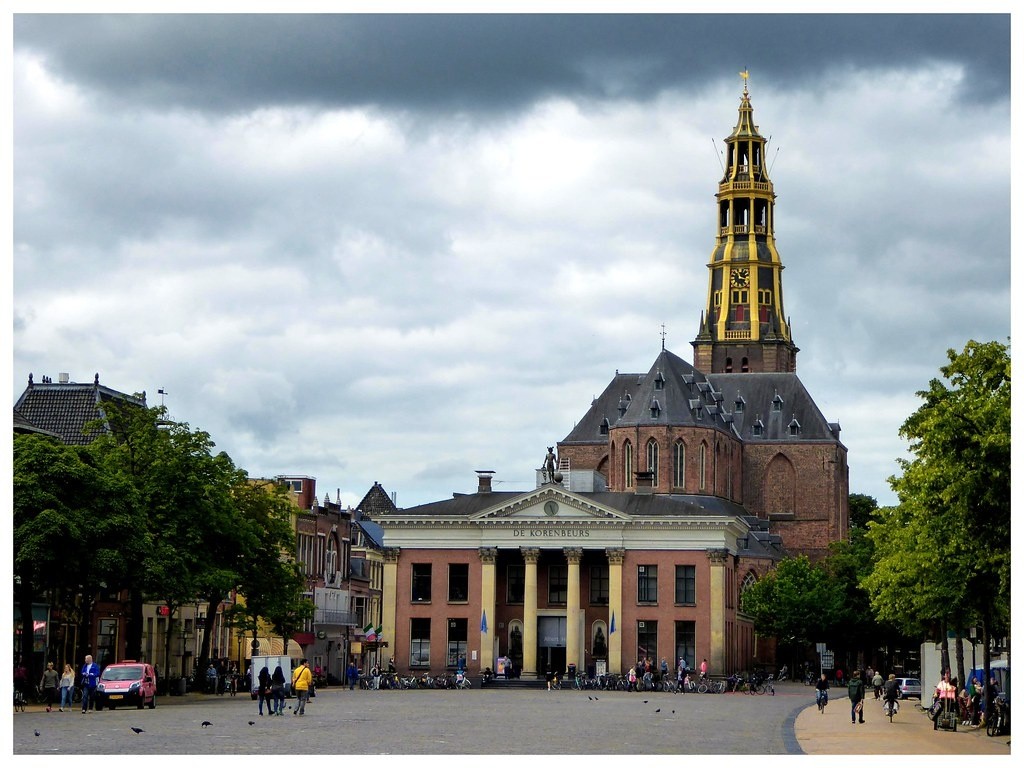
[482, 667, 493, 685]
[936, 670, 998, 729]
[80, 654, 101, 714]
[661, 656, 669, 680]
[154, 663, 163, 678]
[836, 665, 901, 724]
[39, 662, 60, 713]
[543, 446, 559, 484]
[389, 657, 393, 672]
[815, 674, 831, 710]
[510, 625, 522, 652]
[346, 662, 358, 691]
[56, 664, 76, 712]
[543, 662, 558, 691]
[501, 656, 512, 679]
[372, 664, 383, 690]
[207, 660, 252, 694]
[676, 656, 690, 692]
[700, 658, 708, 681]
[257, 659, 313, 716]
[628, 657, 654, 692]
[458, 655, 463, 670]
[14, 653, 27, 692]
[595, 627, 605, 658]
[779, 663, 815, 683]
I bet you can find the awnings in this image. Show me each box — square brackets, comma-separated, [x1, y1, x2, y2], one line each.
[246, 637, 272, 659]
[271, 638, 304, 659]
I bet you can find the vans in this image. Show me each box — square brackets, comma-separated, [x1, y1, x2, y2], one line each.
[94, 659, 156, 710]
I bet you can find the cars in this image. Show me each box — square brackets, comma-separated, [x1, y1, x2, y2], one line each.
[894, 677, 922, 700]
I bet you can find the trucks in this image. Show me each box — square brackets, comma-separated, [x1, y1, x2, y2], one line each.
[251, 654, 293, 701]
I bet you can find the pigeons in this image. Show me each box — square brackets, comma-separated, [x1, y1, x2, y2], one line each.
[248, 721, 255, 726]
[202, 721, 213, 729]
[655, 708, 660, 713]
[35, 729, 41, 737]
[642, 700, 648, 703]
[672, 710, 675, 713]
[131, 727, 145, 735]
[282, 702, 288, 709]
[588, 696, 599, 701]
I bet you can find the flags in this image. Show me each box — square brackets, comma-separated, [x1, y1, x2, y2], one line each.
[481, 613, 488, 634]
[374, 625, 384, 641]
[610, 613, 616, 636]
[363, 623, 377, 640]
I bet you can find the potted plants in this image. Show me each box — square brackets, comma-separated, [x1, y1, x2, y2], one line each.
[169, 669, 187, 695]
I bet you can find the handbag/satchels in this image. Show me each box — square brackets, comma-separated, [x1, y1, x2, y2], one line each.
[632, 675, 636, 682]
[291, 686, 296, 696]
[854, 699, 863, 712]
[266, 690, 272, 694]
[273, 685, 282, 693]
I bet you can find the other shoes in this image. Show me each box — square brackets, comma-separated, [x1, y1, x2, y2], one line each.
[47, 707, 52, 713]
[59, 708, 63, 712]
[82, 710, 86, 713]
[89, 710, 92, 713]
[269, 711, 274, 714]
[860, 720, 864, 723]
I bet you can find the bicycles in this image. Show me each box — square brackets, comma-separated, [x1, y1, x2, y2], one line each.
[817, 689, 829, 714]
[987, 698, 1006, 737]
[71, 681, 84, 703]
[764, 677, 775, 696]
[884, 695, 899, 723]
[357, 669, 472, 691]
[33, 682, 48, 704]
[13, 688, 27, 713]
[570, 669, 766, 696]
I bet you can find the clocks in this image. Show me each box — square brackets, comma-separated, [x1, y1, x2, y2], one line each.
[730, 269, 750, 288]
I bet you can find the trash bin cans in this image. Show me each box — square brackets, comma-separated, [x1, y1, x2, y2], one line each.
[567, 664, 576, 680]
[588, 663, 594, 679]
